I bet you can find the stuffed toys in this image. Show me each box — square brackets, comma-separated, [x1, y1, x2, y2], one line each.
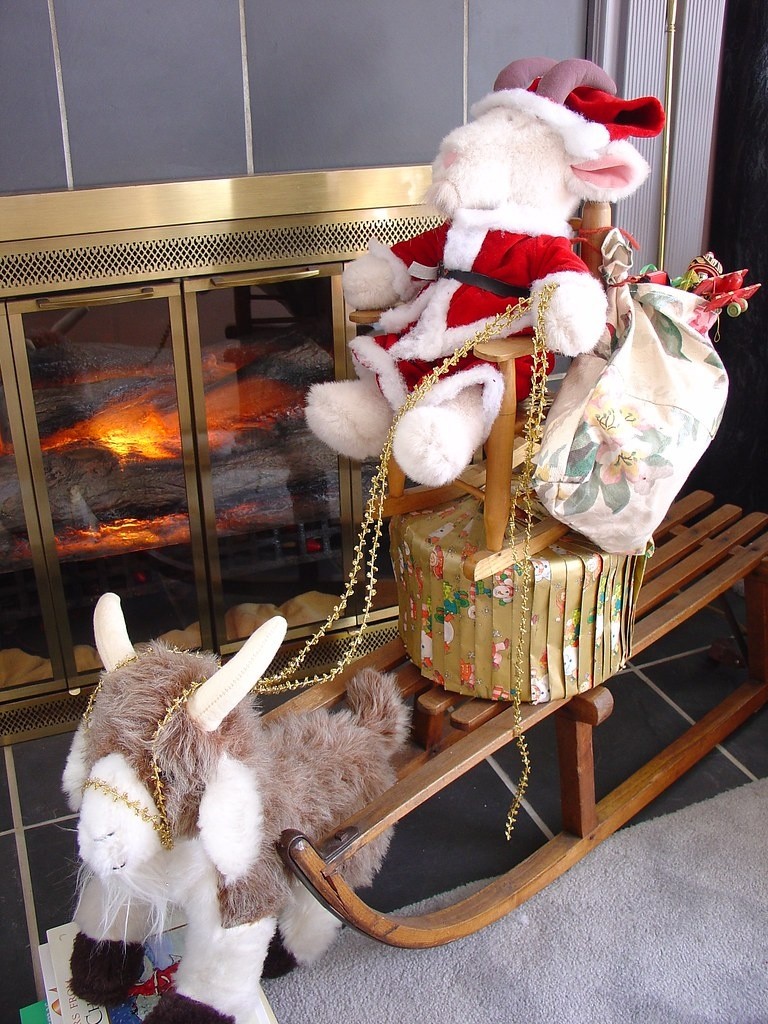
[303, 57, 664, 488]
[69, 592, 411, 1024]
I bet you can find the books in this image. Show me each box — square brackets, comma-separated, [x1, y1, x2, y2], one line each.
[20, 921, 278, 1024]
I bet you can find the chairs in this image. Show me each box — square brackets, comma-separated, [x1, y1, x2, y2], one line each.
[344, 199, 613, 582]
